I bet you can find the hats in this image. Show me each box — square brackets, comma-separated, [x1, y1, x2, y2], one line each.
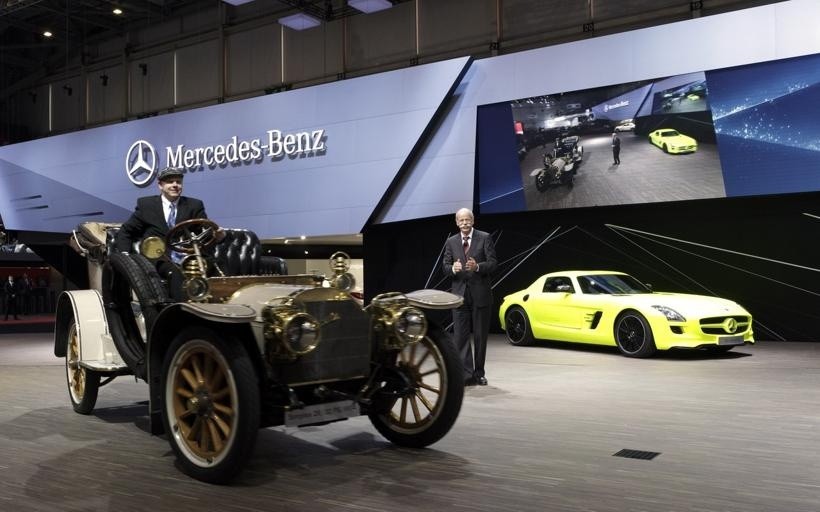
[156, 166, 184, 181]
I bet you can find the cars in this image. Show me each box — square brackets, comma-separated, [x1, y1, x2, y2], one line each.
[614, 122, 635, 133]
[52, 220, 466, 483]
[530, 136, 584, 192]
[687, 93, 700, 102]
[497, 269, 756, 355]
[649, 130, 698, 157]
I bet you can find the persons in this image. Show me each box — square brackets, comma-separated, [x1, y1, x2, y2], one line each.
[679, 97, 681, 106]
[610, 132, 621, 165]
[662, 100, 672, 112]
[554, 137, 567, 157]
[0, 222, 34, 254]
[0, 273, 47, 319]
[442, 208, 498, 385]
[115, 167, 227, 301]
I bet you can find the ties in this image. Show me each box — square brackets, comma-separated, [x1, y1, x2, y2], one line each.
[462, 237, 471, 261]
[167, 202, 177, 230]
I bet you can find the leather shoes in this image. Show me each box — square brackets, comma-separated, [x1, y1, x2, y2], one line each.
[461, 375, 490, 389]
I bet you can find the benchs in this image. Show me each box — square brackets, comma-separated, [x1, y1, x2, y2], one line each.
[93, 213, 291, 299]
